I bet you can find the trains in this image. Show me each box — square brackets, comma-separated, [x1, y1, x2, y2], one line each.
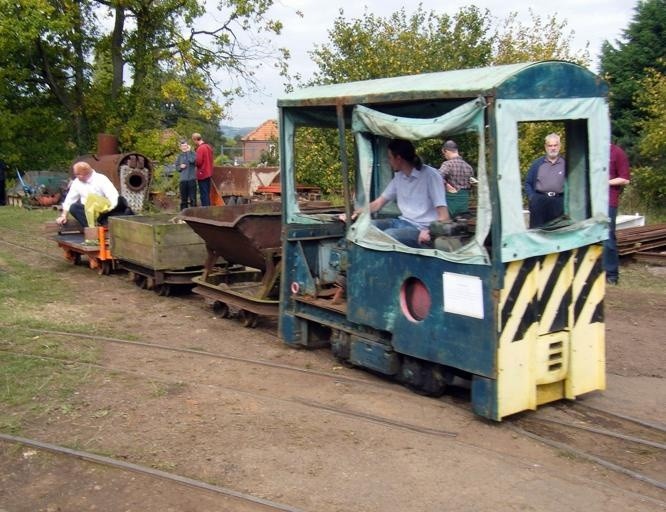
[48, 58, 615, 426]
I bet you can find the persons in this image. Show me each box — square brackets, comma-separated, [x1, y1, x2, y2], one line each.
[524, 133, 567, 230]
[173, 139, 196, 212]
[331, 137, 449, 306]
[191, 133, 213, 206]
[603, 142, 630, 284]
[55, 161, 128, 229]
[436, 139, 475, 218]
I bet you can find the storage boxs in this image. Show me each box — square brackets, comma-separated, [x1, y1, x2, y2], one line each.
[108, 213, 227, 271]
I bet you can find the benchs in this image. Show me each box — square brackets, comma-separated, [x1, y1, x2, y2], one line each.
[457, 214, 573, 267]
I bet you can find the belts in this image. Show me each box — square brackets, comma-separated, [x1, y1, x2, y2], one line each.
[535, 190, 564, 198]
